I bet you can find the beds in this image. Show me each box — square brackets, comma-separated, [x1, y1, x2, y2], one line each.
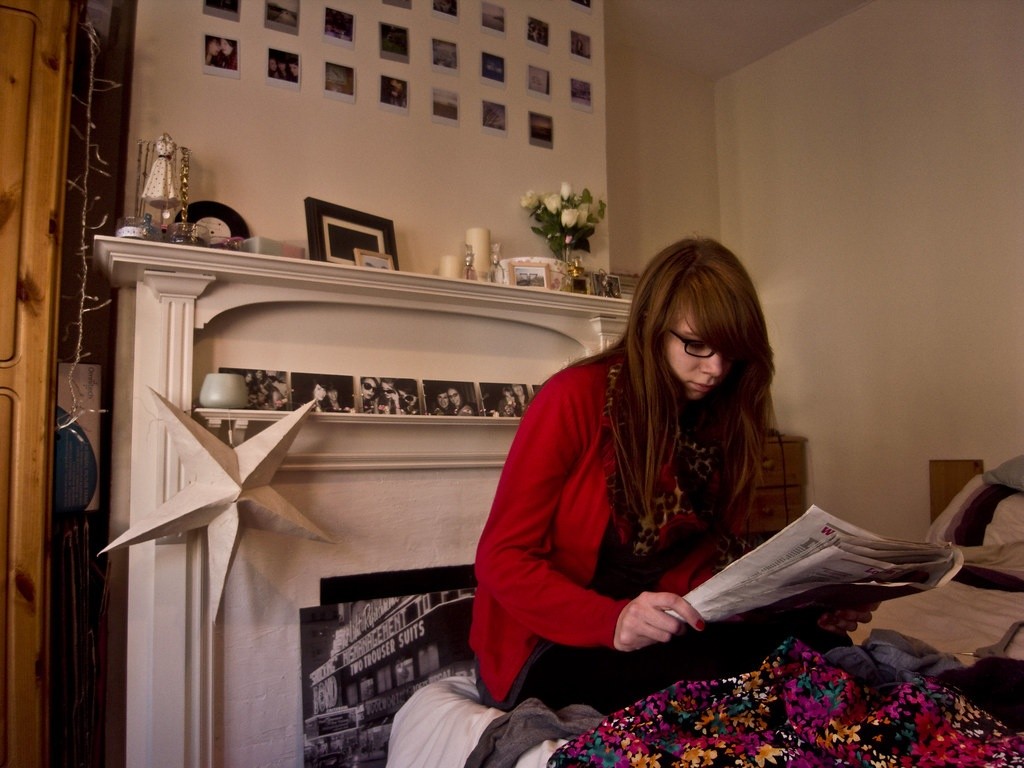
[375, 467, 1024, 768]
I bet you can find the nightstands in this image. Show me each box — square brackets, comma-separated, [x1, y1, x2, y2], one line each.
[741, 435, 808, 544]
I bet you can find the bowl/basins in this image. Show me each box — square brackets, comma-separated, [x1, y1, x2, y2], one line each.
[116, 214, 162, 244]
[199, 374, 250, 409]
[165, 222, 211, 249]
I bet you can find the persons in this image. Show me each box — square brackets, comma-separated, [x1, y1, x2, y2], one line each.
[468, 237, 885, 714]
[204, 36, 236, 70]
[298, 378, 350, 412]
[498, 385, 529, 417]
[245, 371, 288, 411]
[326, 1, 591, 105]
[436, 386, 475, 416]
[269, 54, 300, 81]
[361, 377, 420, 415]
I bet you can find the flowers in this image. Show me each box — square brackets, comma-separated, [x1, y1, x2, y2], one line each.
[518, 187, 608, 287]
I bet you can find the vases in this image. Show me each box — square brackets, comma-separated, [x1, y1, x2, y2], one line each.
[554, 246, 581, 293]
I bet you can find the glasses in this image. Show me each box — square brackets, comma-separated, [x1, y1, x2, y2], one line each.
[667, 327, 716, 358]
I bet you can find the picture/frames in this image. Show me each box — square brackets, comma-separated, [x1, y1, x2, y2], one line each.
[199, 0, 596, 152]
[353, 248, 396, 271]
[304, 197, 400, 271]
[508, 261, 552, 290]
